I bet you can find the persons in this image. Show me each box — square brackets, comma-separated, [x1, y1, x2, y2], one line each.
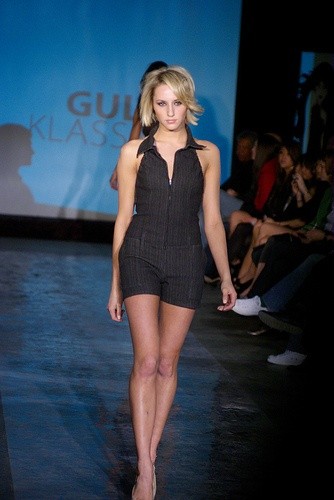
[107, 61, 169, 190]
[206, 124, 333, 385]
[107, 69, 237, 500]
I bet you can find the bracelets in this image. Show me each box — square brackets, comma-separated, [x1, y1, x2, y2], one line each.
[297, 200, 303, 202]
[303, 191, 311, 195]
